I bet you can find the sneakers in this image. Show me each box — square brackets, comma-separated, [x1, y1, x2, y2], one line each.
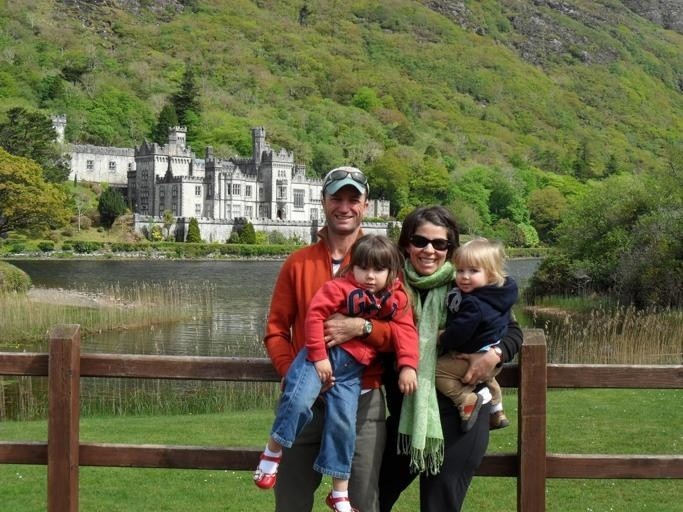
[325, 490, 358, 512]
[254, 450, 282, 490]
[487, 410, 511, 431]
[459, 392, 484, 430]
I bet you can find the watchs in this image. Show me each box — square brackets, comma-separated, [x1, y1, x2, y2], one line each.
[361, 320, 372, 339]
[490, 345, 503, 361]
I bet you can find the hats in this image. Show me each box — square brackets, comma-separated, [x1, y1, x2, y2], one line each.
[323, 166, 368, 195]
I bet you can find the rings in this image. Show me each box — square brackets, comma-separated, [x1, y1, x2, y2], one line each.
[477, 380, 483, 384]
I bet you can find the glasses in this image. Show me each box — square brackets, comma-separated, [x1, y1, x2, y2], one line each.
[411, 234, 449, 251]
[323, 170, 368, 190]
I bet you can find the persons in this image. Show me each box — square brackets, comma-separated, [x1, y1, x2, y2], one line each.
[434, 237, 518, 433]
[263, 166, 386, 511]
[377, 204, 523, 510]
[252, 234, 418, 510]
